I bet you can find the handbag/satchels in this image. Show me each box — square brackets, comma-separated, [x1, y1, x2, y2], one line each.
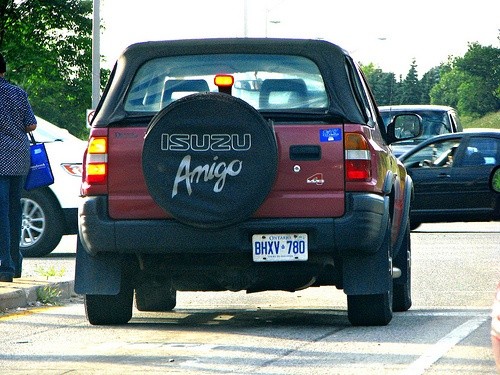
[24, 131, 55, 192]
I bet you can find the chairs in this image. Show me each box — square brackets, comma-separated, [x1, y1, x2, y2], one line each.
[161, 79, 210, 109]
[260, 79, 308, 108]
[469, 152, 486, 166]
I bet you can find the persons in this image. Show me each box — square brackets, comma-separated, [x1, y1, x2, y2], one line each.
[0, 52, 37, 282]
[424, 146, 457, 168]
[399, 127, 414, 138]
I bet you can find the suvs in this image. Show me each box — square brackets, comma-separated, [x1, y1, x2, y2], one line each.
[74, 38, 415, 326]
[377, 104, 463, 135]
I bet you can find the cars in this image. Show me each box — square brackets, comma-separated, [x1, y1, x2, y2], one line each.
[19, 115, 88, 257]
[389, 130, 500, 230]
